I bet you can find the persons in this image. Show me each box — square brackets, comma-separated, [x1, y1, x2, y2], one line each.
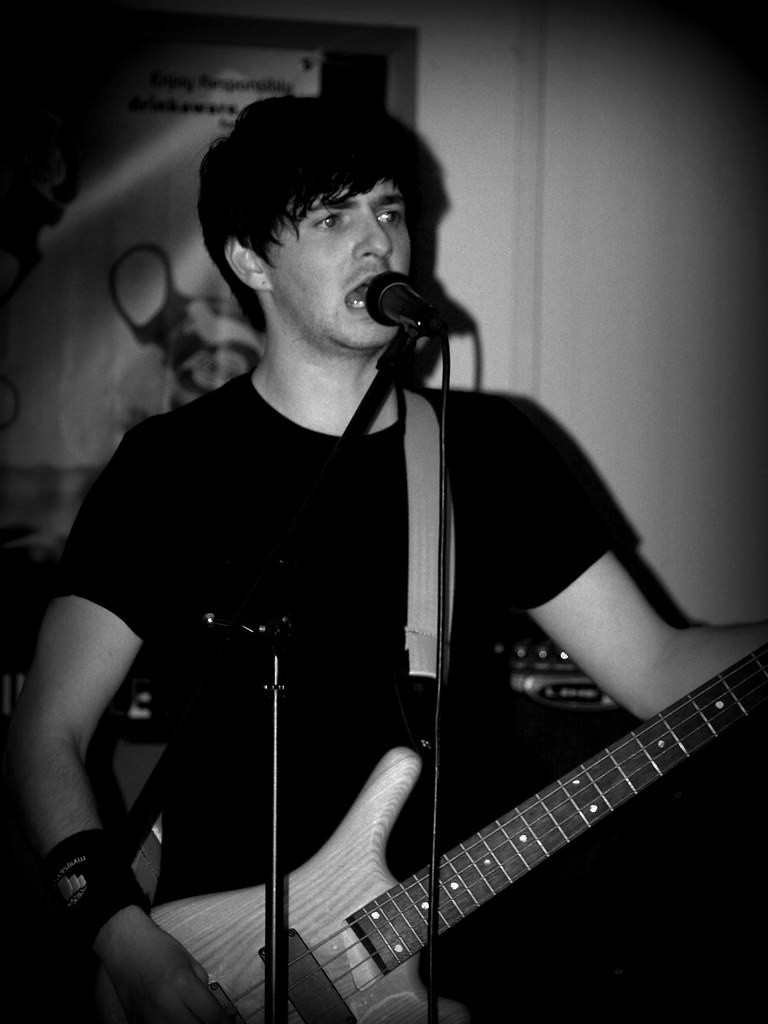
[0, 98, 768, 1023]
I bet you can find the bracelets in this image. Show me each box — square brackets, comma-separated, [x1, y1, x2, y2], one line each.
[39, 830, 149, 952]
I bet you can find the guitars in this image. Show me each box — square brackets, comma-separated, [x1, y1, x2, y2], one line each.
[149, 641, 768, 1023]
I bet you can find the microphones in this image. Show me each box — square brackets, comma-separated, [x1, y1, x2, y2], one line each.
[364, 271, 445, 335]
